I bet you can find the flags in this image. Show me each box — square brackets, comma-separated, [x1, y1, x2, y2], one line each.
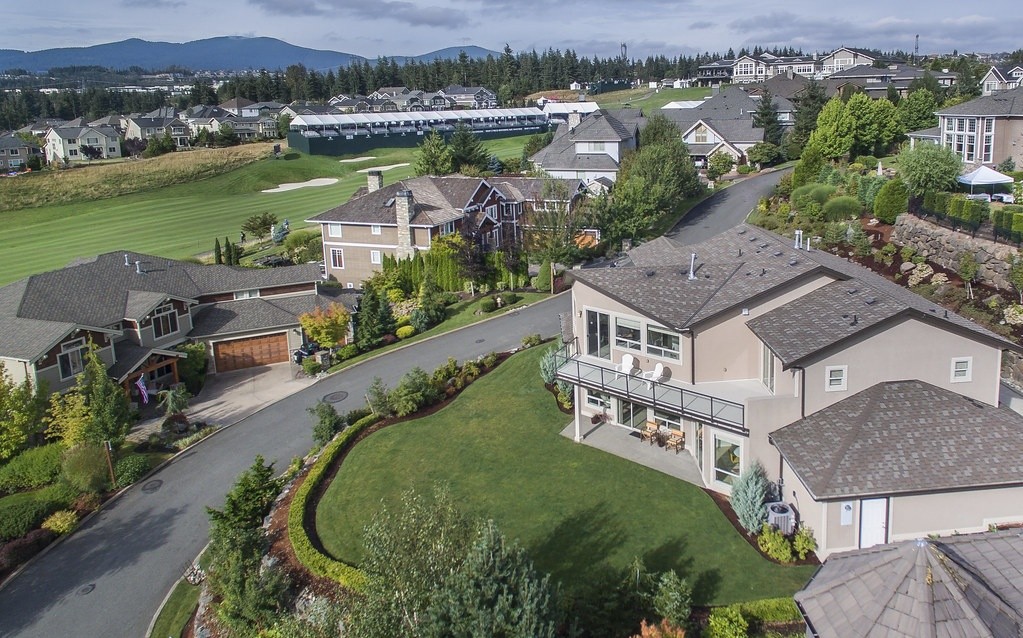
[136, 373, 149, 404]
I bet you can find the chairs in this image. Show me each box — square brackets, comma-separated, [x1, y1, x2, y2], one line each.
[665, 428, 685, 455]
[641, 421, 659, 446]
[728, 449, 740, 465]
[615, 354, 635, 381]
[641, 362, 664, 391]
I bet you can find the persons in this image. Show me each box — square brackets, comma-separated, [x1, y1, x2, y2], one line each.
[297, 344, 308, 366]
[284, 218, 289, 229]
[159, 383, 165, 391]
[701, 157, 706, 169]
[240, 230, 247, 245]
[270, 225, 276, 237]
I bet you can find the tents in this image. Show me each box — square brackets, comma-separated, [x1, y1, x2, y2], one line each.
[290, 106, 545, 136]
[956, 164, 1015, 196]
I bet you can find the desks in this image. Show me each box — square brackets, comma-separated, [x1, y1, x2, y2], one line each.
[966, 193, 1014, 204]
[734, 447, 740, 458]
